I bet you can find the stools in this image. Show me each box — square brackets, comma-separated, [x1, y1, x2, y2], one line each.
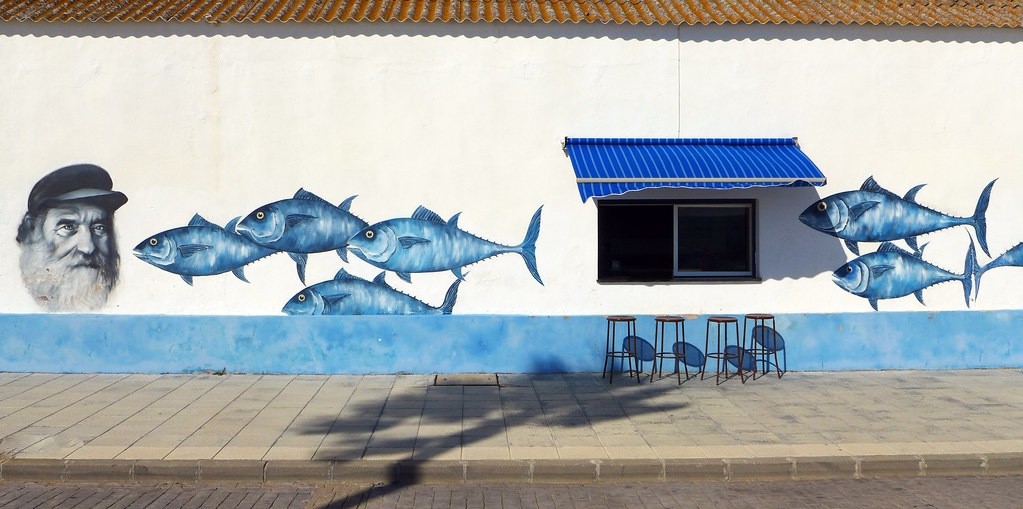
[649, 317, 690, 386]
[700, 316, 745, 385]
[602, 316, 641, 384]
[738, 313, 781, 381]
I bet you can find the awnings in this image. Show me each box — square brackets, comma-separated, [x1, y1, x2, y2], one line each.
[559, 135, 828, 203]
